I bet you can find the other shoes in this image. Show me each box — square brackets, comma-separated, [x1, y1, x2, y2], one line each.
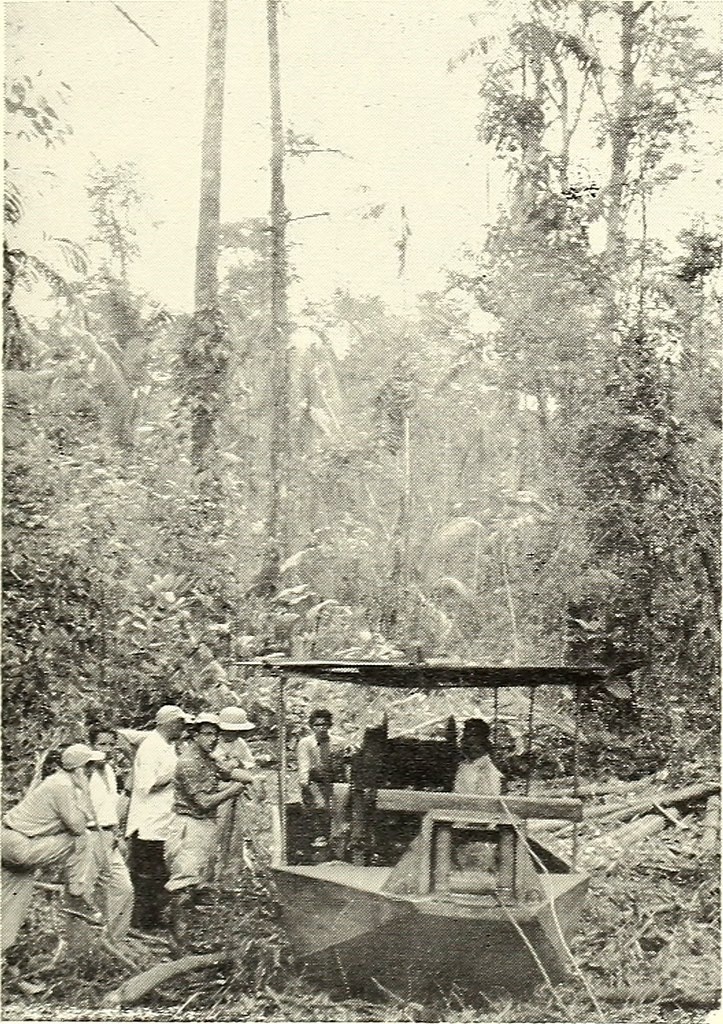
[61, 895, 103, 925]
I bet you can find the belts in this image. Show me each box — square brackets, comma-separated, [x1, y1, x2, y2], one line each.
[86, 826, 113, 831]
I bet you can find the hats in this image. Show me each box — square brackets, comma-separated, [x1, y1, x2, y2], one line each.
[61, 744, 106, 769]
[217, 707, 255, 730]
[194, 711, 220, 724]
[155, 705, 195, 724]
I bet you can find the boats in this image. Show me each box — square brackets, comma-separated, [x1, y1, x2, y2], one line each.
[262, 654, 607, 1006]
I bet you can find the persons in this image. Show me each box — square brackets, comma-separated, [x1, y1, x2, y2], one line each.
[1, 743, 105, 952]
[452, 719, 505, 826]
[212, 707, 272, 845]
[54, 729, 133, 962]
[124, 704, 192, 928]
[166, 712, 247, 896]
[298, 710, 352, 845]
